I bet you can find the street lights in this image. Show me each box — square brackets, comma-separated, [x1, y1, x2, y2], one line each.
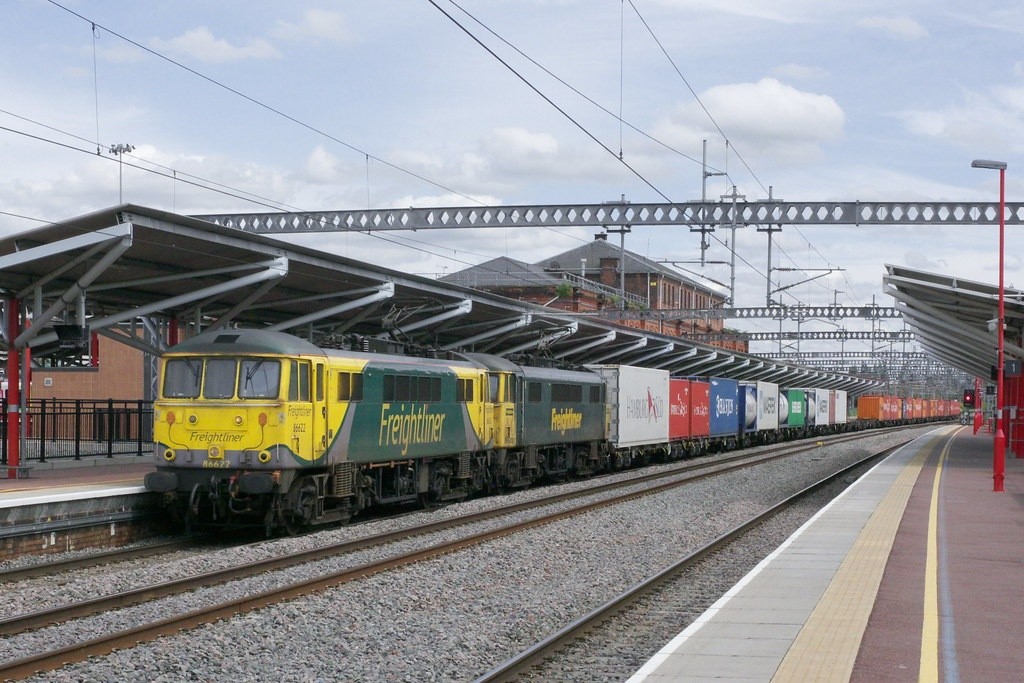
[970, 158, 1007, 492]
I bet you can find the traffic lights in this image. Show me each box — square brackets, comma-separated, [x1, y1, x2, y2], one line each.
[963, 388, 974, 407]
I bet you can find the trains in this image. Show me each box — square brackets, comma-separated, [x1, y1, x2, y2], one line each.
[143, 327, 961, 539]
